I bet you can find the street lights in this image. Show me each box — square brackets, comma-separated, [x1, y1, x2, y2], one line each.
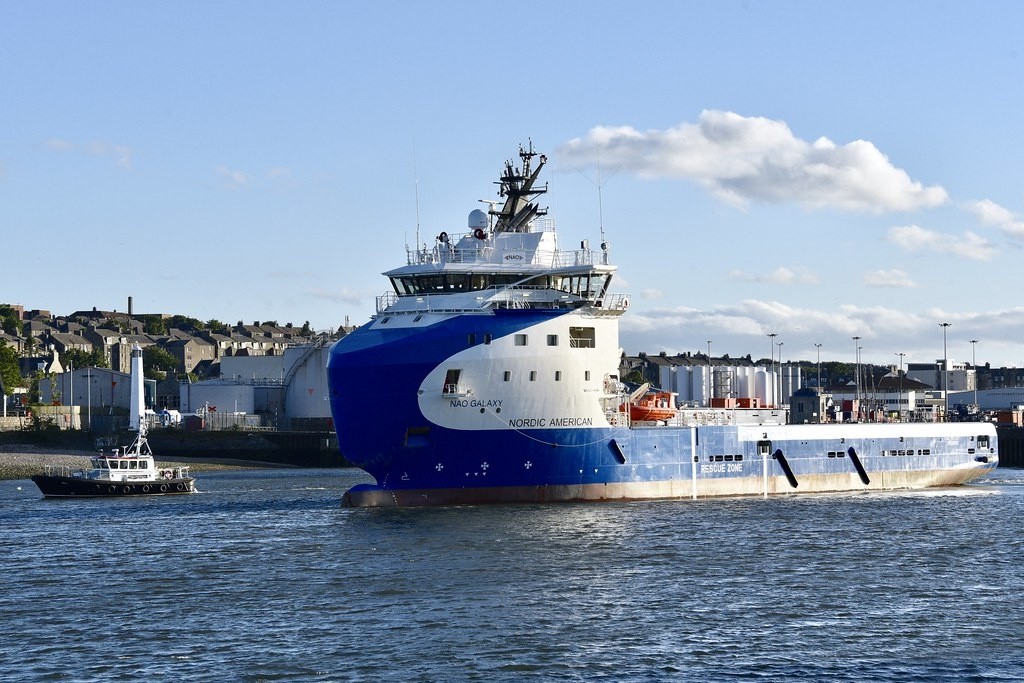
[707, 340, 712, 398]
[858, 347, 864, 398]
[814, 343, 823, 392]
[938, 322, 952, 416]
[766, 332, 778, 404]
[894, 352, 906, 423]
[775, 342, 783, 404]
[852, 336, 862, 399]
[969, 340, 980, 404]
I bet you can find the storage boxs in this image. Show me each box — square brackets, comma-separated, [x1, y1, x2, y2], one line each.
[826, 399, 897, 424]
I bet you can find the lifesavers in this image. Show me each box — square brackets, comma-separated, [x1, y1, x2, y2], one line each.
[123, 486, 130, 494]
[94, 485, 100, 491]
[160, 485, 167, 492]
[177, 483, 183, 490]
[474, 229, 483, 238]
[440, 232, 447, 242]
[165, 471, 171, 478]
[622, 297, 629, 308]
[142, 485, 149, 493]
[108, 485, 116, 493]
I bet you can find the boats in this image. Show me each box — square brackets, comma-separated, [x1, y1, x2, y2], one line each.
[31, 413, 201, 500]
[324, 134, 1001, 511]
[618, 392, 680, 421]
